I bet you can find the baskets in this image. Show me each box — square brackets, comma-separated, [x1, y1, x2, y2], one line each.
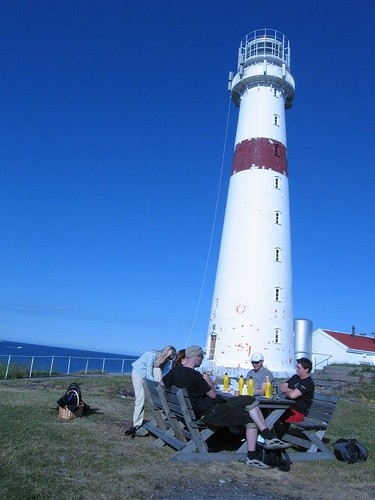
[58, 390, 84, 421]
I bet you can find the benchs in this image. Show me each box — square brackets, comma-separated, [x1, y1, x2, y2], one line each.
[134, 378, 221, 454]
[278, 387, 340, 454]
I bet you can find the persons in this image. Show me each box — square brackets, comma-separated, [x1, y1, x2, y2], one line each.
[274, 357, 315, 428]
[131, 346, 176, 436]
[160, 344, 292, 469]
[244, 352, 273, 395]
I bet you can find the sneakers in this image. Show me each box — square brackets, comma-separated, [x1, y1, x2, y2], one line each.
[265, 438, 292, 449]
[246, 458, 272, 469]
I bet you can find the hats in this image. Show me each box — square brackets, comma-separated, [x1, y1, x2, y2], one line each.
[185, 345, 206, 357]
[250, 351, 265, 362]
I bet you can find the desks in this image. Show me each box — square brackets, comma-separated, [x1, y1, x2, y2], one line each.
[170, 386, 337, 464]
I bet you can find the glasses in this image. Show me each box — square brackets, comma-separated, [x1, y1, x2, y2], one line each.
[252, 361, 261, 364]
[167, 357, 173, 360]
[296, 366, 305, 371]
[199, 355, 204, 359]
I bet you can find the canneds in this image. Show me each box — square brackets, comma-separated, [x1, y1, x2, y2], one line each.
[272, 385, 278, 395]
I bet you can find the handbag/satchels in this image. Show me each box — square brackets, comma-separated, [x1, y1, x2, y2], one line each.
[332, 437, 370, 463]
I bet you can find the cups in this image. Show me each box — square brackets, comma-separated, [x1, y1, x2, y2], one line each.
[234, 390, 239, 396]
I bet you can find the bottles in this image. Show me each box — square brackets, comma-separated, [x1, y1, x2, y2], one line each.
[239, 375, 244, 396]
[248, 376, 253, 396]
[264, 374, 271, 399]
[273, 383, 279, 395]
[224, 373, 228, 390]
